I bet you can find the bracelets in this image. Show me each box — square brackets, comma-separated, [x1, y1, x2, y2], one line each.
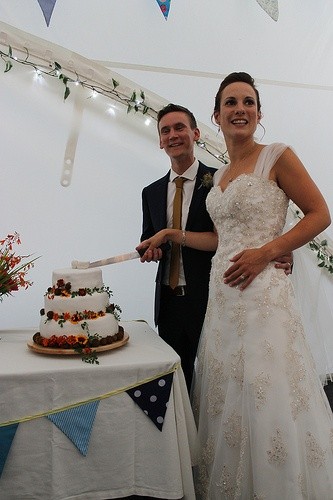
[182, 230, 186, 246]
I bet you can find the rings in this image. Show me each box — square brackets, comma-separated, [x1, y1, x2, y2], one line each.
[240, 276, 245, 279]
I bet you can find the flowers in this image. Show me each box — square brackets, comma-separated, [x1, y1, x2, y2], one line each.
[33, 330, 103, 366]
[195, 171, 214, 191]
[43, 279, 114, 301]
[0, 230, 43, 304]
[39, 304, 123, 329]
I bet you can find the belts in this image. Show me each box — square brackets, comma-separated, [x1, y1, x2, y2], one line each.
[160, 284, 188, 297]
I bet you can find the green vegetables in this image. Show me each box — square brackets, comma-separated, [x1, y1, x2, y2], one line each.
[73, 346, 99, 365]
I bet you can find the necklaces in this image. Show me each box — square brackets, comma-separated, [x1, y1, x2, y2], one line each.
[228, 161, 234, 182]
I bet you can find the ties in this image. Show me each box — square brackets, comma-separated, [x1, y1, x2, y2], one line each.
[169, 177, 189, 290]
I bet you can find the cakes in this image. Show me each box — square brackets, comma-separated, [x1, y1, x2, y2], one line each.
[33, 256, 124, 349]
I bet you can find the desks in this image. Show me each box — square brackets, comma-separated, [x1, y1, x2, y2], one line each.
[0, 319, 201, 500]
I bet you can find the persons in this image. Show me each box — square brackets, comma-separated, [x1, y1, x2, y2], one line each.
[141, 102, 293, 392]
[136, 72, 333, 500]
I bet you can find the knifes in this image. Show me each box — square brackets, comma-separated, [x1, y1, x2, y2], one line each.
[89, 246, 149, 268]
